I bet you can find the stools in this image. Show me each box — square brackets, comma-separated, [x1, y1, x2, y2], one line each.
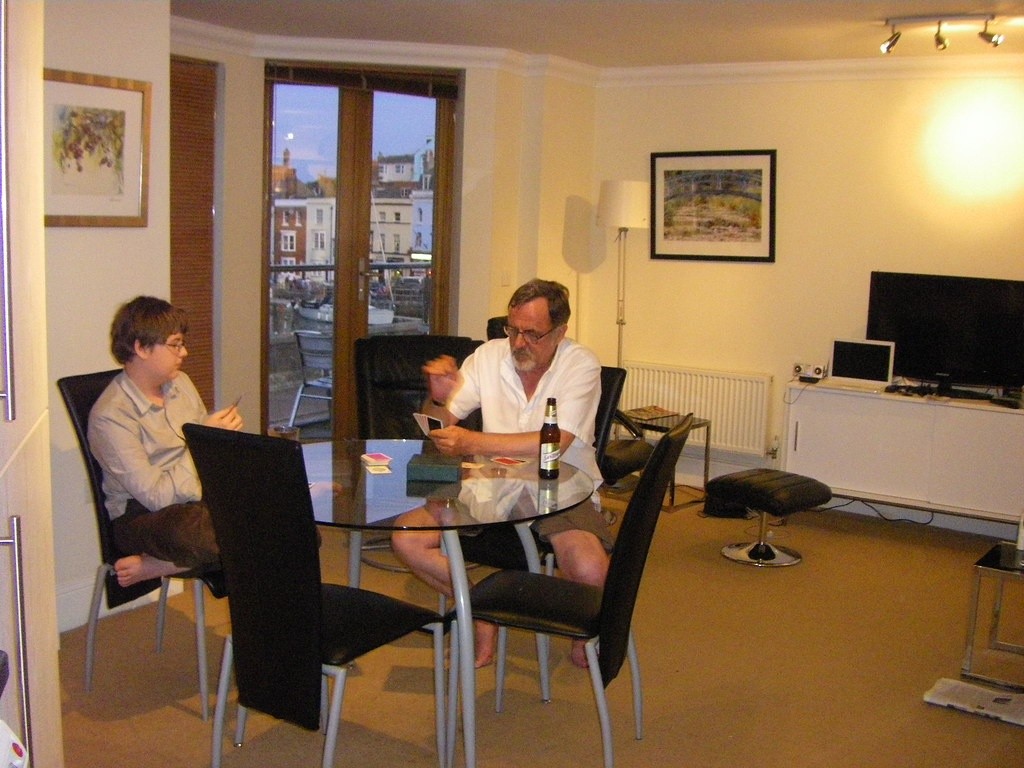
[614, 405, 711, 514]
[705, 468, 833, 569]
[960, 543, 1024, 694]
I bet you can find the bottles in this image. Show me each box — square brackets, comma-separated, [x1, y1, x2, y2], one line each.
[538, 398, 561, 481]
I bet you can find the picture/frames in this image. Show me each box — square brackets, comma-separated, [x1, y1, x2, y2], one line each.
[650, 148, 778, 260]
[42, 67, 152, 229]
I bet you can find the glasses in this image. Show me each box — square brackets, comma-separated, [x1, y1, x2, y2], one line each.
[503, 320, 557, 345]
[154, 339, 188, 354]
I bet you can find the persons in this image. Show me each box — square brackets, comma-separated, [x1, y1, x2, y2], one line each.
[85, 295, 320, 571]
[390, 280, 614, 670]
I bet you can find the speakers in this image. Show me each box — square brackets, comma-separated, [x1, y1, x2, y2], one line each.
[792, 363, 825, 378]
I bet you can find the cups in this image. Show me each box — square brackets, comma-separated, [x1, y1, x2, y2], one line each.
[270, 427, 301, 440]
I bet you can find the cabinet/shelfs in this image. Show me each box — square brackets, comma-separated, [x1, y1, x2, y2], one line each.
[780, 378, 1024, 549]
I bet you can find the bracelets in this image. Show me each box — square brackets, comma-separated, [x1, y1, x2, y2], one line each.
[432, 397, 445, 407]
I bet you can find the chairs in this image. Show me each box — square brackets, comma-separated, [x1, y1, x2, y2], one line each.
[55, 368, 226, 719]
[444, 411, 692, 768]
[460, 368, 625, 703]
[288, 330, 333, 428]
[183, 421, 447, 768]
[348, 331, 486, 594]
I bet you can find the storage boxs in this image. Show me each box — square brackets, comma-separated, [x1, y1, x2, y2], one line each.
[407, 454, 462, 482]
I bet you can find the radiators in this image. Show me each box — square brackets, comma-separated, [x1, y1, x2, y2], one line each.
[622, 361, 772, 457]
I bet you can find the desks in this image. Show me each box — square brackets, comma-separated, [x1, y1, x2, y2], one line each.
[298, 435, 595, 768]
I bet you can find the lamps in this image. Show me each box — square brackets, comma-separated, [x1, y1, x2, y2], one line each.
[596, 180, 651, 438]
[879, 14, 1005, 54]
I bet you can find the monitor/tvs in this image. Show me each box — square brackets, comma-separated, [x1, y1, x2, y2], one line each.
[866, 271, 1024, 400]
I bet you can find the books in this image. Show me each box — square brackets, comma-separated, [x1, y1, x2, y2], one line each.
[625, 405, 678, 420]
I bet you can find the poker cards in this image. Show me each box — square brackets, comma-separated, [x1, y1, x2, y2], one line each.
[491, 455, 529, 469]
[360, 452, 393, 475]
[412, 413, 444, 438]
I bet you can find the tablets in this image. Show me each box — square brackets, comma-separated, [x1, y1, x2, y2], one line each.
[827, 337, 895, 388]
